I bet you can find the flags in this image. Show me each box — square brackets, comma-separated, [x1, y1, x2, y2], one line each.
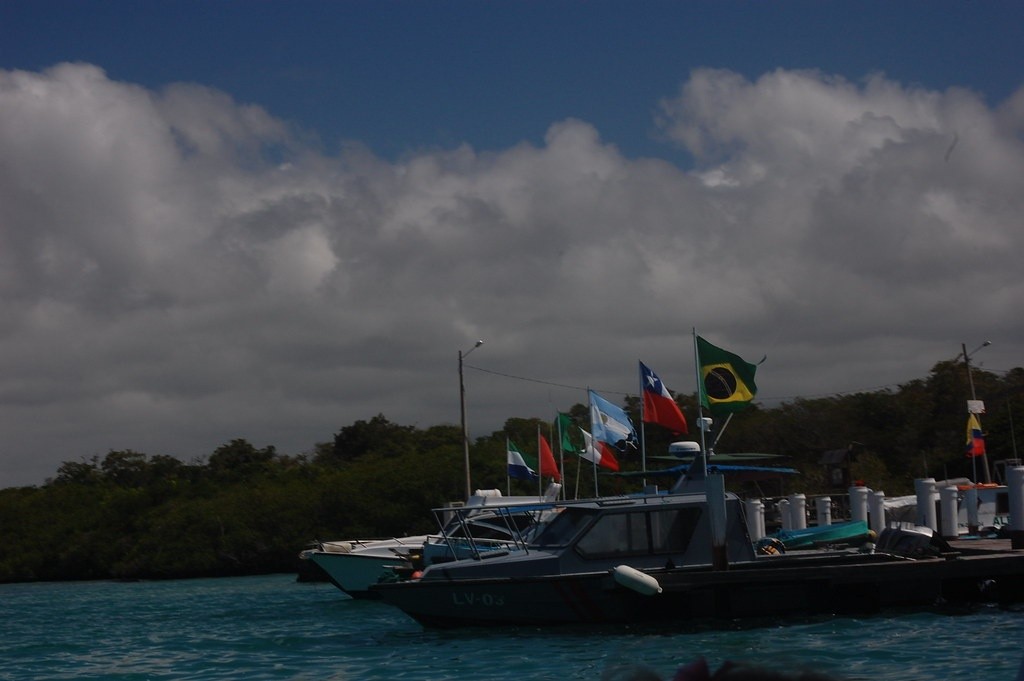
[589, 389, 638, 451]
[966, 415, 985, 457]
[508, 440, 536, 480]
[559, 414, 620, 470]
[697, 334, 767, 412]
[539, 436, 560, 480]
[639, 360, 688, 435]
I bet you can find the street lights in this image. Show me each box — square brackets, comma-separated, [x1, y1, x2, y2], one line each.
[964, 341, 993, 484]
[457, 339, 485, 499]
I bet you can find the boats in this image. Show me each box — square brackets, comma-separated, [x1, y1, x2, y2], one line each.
[300, 326, 962, 638]
